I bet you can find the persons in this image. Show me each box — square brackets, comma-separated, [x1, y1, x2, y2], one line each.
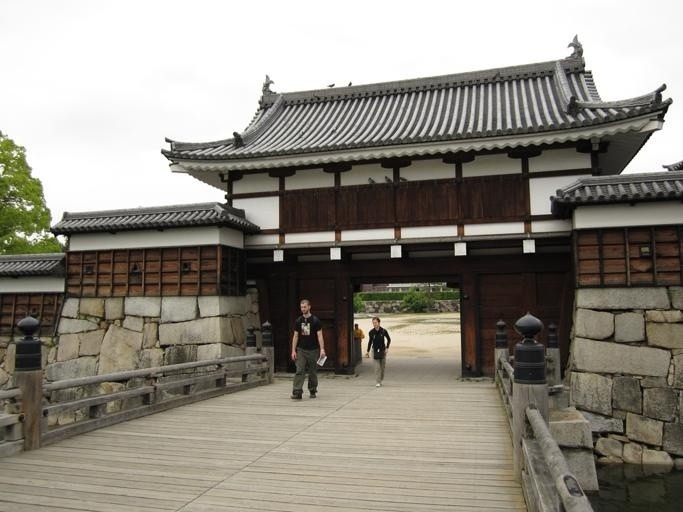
[354, 324, 365, 340]
[365, 317, 391, 386]
[290, 299, 326, 399]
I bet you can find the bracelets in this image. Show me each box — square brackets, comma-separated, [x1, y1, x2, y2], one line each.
[321, 349, 324, 352]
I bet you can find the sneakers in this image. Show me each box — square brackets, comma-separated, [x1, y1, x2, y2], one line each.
[290, 393, 302, 399]
[375, 382, 381, 387]
[308, 391, 316, 398]
